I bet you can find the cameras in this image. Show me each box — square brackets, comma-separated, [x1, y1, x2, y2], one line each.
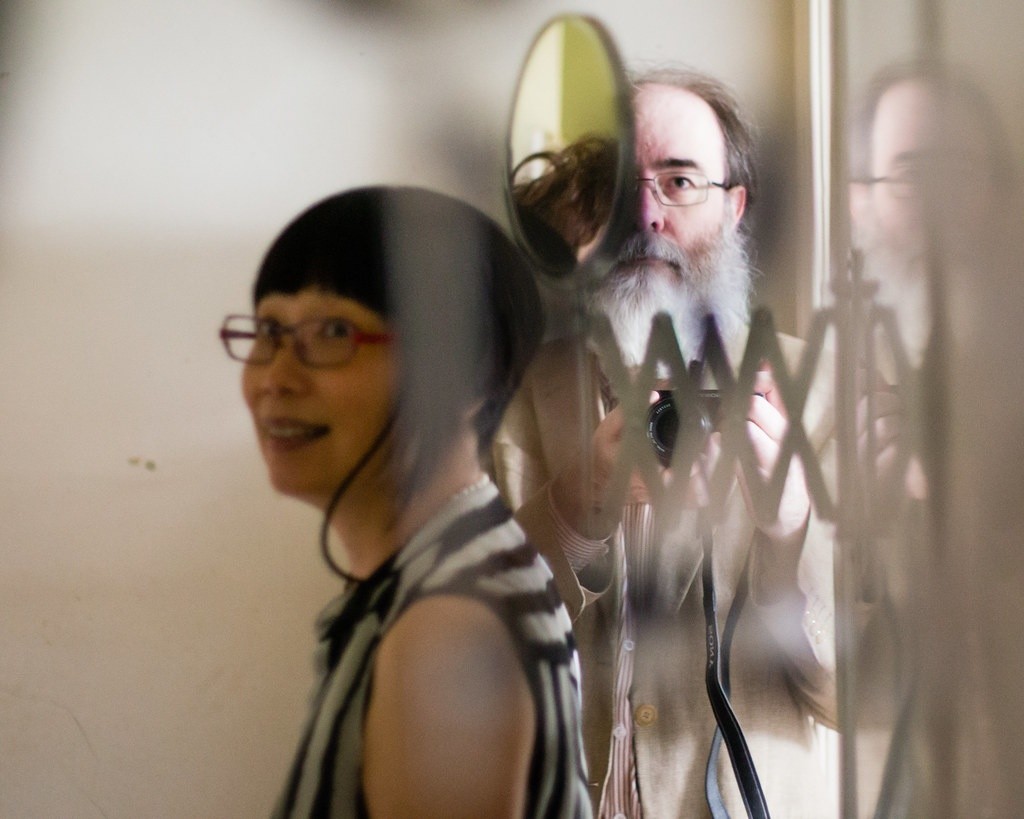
[645, 386, 763, 469]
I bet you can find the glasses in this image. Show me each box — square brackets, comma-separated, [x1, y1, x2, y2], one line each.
[218, 314, 393, 365]
[633, 172, 727, 208]
[844, 164, 923, 195]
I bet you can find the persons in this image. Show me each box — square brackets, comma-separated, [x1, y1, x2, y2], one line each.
[850, 63, 1006, 509]
[219, 186, 590, 819]
[493, 66, 837, 819]
